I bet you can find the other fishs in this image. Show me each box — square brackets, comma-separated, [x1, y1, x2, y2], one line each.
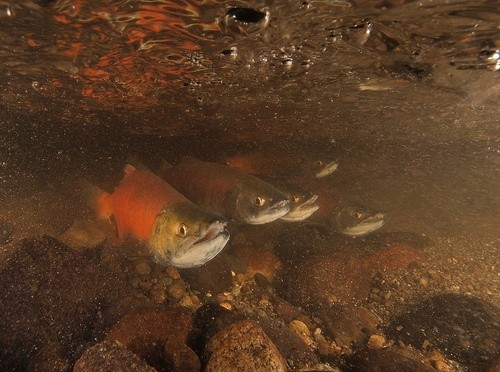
[65, 149, 385, 271]
